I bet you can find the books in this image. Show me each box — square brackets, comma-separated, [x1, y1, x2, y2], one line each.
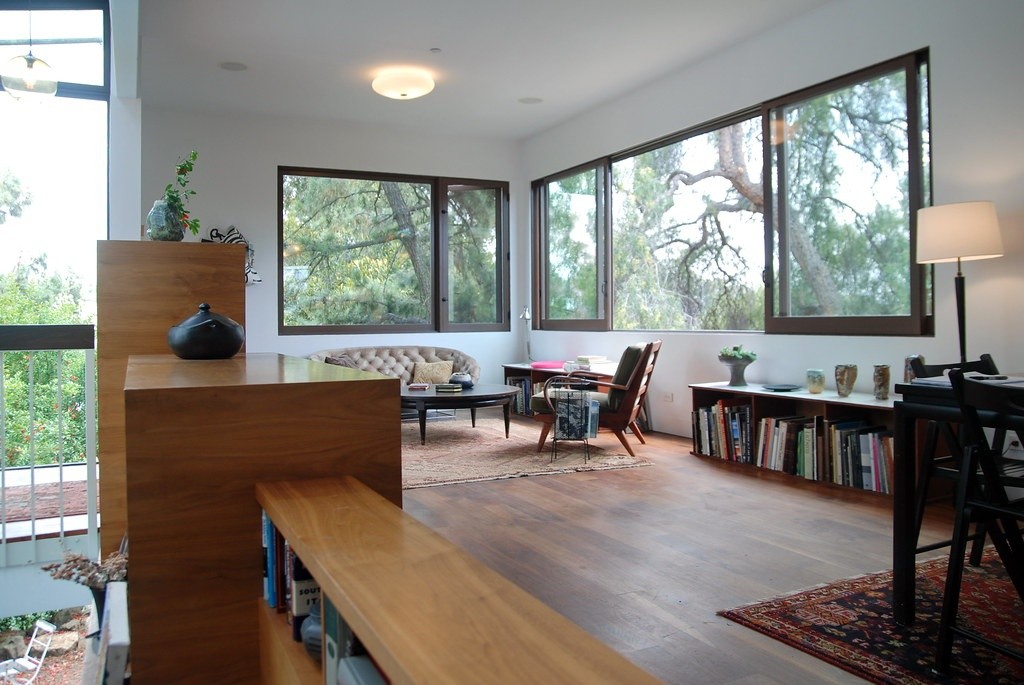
[407, 385, 429, 390]
[261, 509, 320, 643]
[507, 376, 599, 440]
[691, 396, 894, 495]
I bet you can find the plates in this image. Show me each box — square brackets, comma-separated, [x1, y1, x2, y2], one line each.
[761, 384, 801, 392]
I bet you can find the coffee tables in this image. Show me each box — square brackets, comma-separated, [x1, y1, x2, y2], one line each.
[400, 382, 520, 445]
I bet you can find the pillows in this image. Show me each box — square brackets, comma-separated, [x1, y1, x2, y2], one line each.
[413, 360, 453, 384]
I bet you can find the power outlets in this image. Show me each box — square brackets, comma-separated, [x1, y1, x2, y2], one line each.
[663, 390, 673, 402]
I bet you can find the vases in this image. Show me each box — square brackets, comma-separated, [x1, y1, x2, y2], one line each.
[146, 201, 186, 241]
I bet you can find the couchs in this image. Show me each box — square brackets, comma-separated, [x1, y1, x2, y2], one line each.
[309, 346, 482, 422]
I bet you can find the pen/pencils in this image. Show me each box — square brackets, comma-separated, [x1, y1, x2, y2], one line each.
[969, 375, 1008, 380]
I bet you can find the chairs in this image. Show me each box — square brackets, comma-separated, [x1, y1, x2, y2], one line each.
[0, 619, 55, 685]
[879, 353, 1024, 685]
[533, 336, 662, 456]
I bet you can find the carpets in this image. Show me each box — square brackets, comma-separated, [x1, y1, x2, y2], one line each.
[717, 541, 1024, 685]
[402, 415, 655, 491]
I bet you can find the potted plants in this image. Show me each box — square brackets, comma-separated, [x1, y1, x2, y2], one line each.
[718, 344, 757, 386]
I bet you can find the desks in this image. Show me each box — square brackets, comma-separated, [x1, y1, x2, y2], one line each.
[889, 372, 1024, 622]
[502, 360, 651, 433]
[691, 382, 955, 497]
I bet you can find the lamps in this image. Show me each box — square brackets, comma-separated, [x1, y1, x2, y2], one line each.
[372, 73, 437, 101]
[916, 198, 1007, 382]
[0, 9, 58, 104]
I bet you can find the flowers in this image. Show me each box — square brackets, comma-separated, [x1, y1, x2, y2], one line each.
[159, 148, 209, 233]
